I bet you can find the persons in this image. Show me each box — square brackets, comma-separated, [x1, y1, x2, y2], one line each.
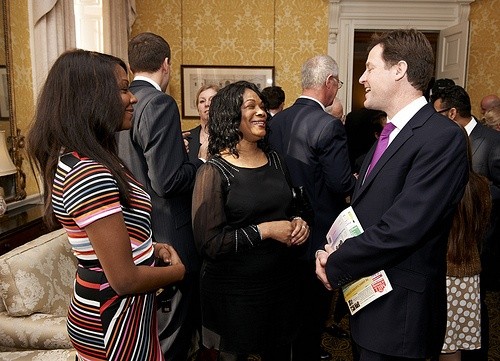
[181, 83, 221, 174]
[437, 172, 492, 361]
[267, 56, 359, 361]
[191, 79, 311, 361]
[322, 79, 500, 361]
[26, 50, 185, 361]
[117, 32, 209, 361]
[316, 28, 472, 361]
[258, 86, 285, 122]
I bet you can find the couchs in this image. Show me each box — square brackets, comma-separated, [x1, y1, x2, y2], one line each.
[0, 228, 78, 361]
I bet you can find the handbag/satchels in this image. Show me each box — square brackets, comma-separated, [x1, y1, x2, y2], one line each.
[153, 265, 184, 354]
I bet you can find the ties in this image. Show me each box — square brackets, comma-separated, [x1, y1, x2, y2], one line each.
[362, 123, 396, 188]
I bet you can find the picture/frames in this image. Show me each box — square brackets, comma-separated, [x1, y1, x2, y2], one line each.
[180, 64, 275, 120]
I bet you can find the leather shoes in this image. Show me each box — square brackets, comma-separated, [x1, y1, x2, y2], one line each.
[320, 348, 332, 360]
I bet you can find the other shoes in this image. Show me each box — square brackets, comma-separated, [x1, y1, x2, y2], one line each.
[326, 324, 349, 339]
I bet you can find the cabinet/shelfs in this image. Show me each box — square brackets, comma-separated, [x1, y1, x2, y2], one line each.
[0, 202, 47, 257]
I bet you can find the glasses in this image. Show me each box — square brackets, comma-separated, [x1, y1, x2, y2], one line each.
[332, 76, 343, 89]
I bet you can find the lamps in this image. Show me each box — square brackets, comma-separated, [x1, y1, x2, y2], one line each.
[0, 129, 17, 216]
